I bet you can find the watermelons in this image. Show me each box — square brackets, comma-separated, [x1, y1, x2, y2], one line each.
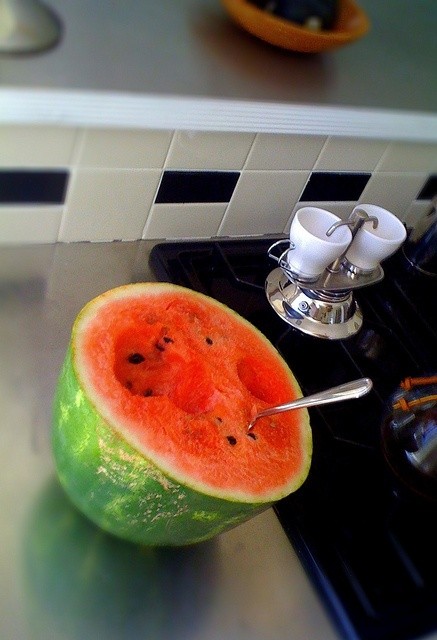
[48, 281, 313, 549]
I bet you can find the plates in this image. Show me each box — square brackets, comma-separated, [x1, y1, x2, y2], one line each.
[220, 0, 370, 53]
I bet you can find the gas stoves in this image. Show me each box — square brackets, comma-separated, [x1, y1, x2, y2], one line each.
[150, 219, 437, 640]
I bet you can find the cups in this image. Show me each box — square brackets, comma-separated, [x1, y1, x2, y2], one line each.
[287, 206, 352, 278]
[345, 203, 407, 271]
